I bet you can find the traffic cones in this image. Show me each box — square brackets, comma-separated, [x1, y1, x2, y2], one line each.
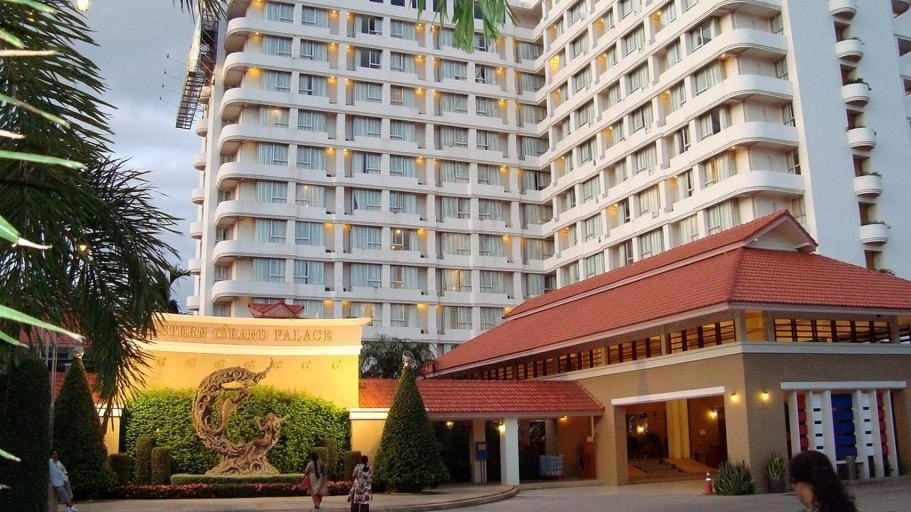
[703, 470, 715, 494]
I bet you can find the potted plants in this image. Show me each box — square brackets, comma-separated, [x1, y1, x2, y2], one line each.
[761, 452, 788, 493]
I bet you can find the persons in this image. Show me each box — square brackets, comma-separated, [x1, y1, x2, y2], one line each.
[47, 446, 80, 511]
[787, 449, 860, 511]
[347, 454, 373, 512]
[303, 451, 328, 511]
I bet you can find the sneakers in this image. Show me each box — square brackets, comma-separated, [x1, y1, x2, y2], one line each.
[63, 507, 79, 512]
[313, 505, 321, 512]
